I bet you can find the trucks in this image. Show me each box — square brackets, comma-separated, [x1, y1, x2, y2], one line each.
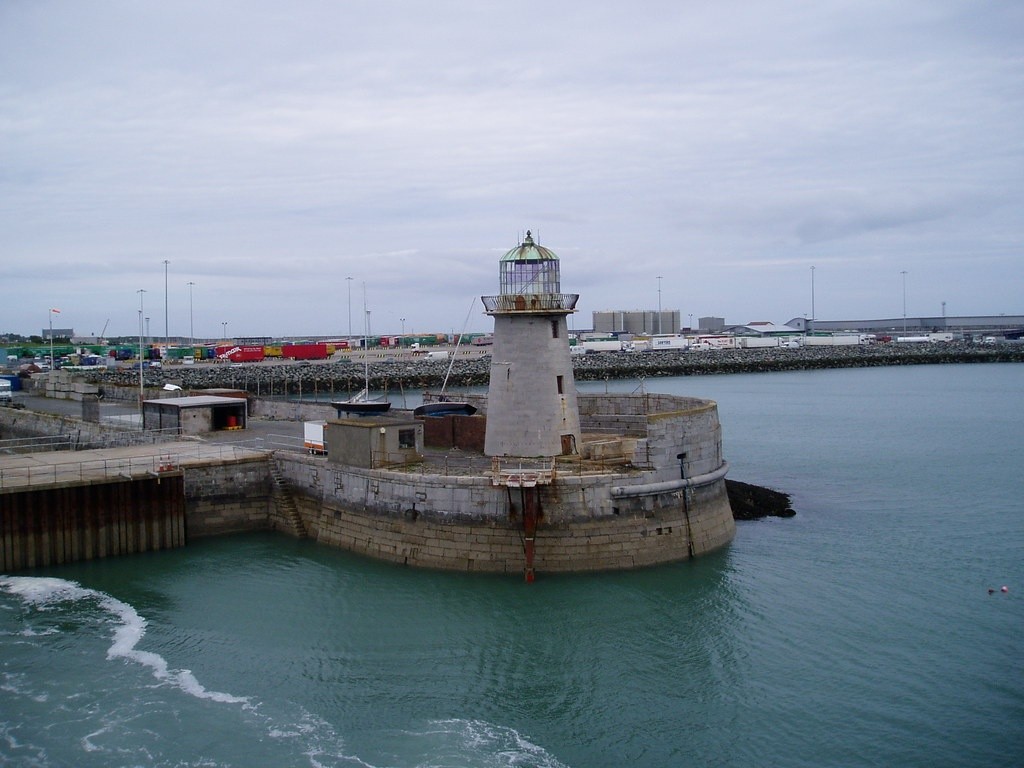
[625, 343, 646, 353]
[688, 343, 709, 352]
[423, 351, 449, 361]
[411, 343, 429, 356]
[780, 342, 798, 348]
[568, 346, 585, 355]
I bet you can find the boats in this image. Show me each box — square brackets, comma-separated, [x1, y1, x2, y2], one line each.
[331, 389, 391, 414]
[413, 401, 477, 421]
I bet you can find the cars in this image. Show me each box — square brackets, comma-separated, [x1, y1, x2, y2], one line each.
[985, 337, 995, 343]
